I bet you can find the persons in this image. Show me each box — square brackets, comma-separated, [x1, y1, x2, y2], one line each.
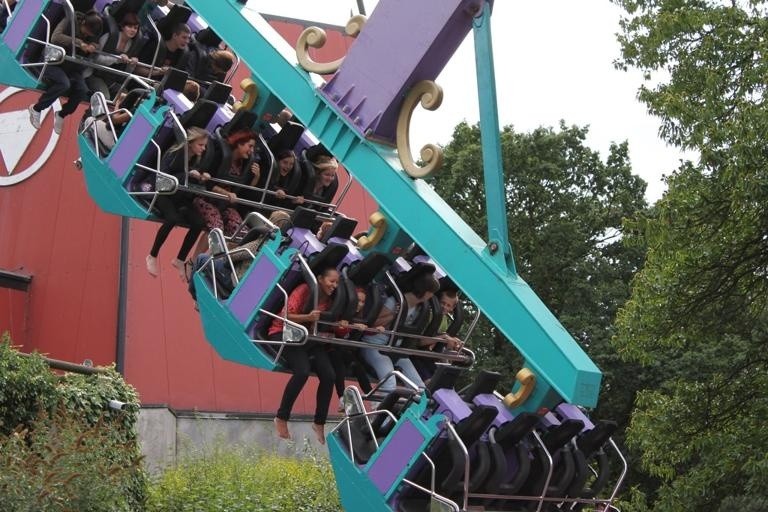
[266, 267, 459, 444]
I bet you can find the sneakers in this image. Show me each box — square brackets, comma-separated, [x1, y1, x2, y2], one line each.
[53, 110, 64, 135]
[28, 104, 42, 130]
